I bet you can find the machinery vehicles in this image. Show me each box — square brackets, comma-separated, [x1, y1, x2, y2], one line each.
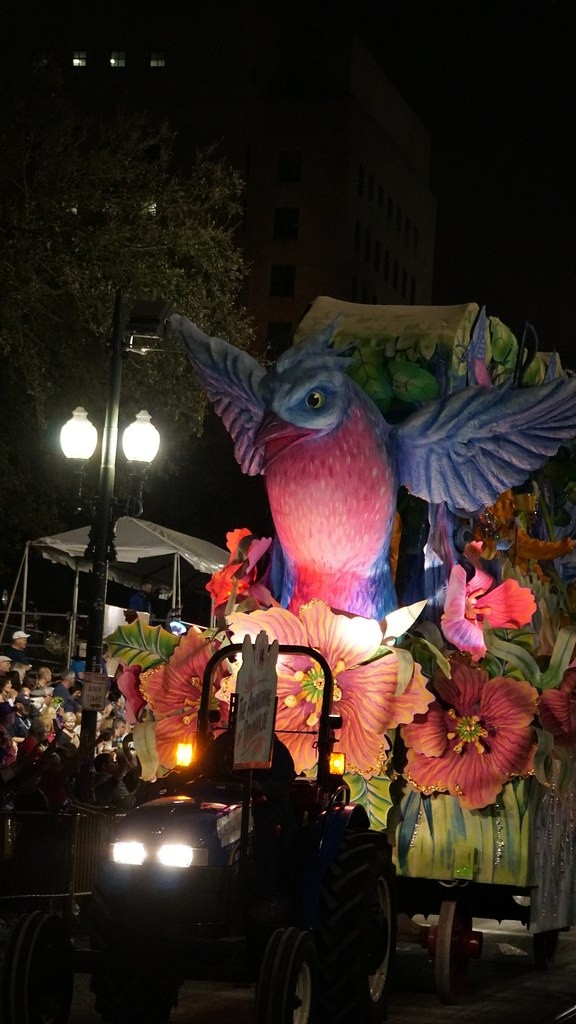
[1, 643, 399, 1023]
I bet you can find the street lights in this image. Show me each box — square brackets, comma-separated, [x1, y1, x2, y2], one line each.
[57, 405, 163, 812]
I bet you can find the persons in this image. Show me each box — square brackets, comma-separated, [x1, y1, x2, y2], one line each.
[186, 731, 296, 922]
[131, 582, 152, 612]
[0, 631, 175, 845]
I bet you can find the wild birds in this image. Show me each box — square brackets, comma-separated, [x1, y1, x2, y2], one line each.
[173, 314, 576, 624]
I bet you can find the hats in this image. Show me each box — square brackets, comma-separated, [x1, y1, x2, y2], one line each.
[14, 694, 34, 704]
[0, 701, 18, 715]
[0, 656, 12, 664]
[12, 631, 30, 639]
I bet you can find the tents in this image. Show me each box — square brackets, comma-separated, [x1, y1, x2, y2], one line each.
[21, 517, 233, 666]
